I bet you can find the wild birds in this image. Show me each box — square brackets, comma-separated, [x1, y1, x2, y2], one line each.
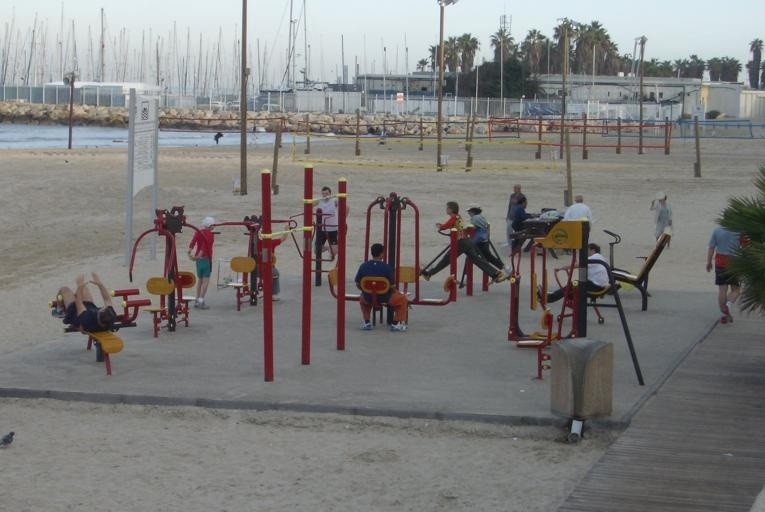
[0, 431, 16, 447]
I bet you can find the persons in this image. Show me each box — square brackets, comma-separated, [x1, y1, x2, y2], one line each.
[707, 209, 752, 323]
[258, 225, 290, 301]
[51, 271, 119, 332]
[354, 243, 409, 331]
[650, 193, 673, 247]
[188, 217, 217, 310]
[313, 187, 350, 261]
[420, 183, 611, 304]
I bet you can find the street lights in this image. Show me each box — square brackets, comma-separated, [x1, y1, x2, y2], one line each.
[63, 71, 81, 149]
[434, 0, 461, 171]
[633, 34, 649, 156]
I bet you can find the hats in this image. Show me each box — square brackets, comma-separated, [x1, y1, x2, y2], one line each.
[202, 217, 216, 227]
[466, 203, 482, 211]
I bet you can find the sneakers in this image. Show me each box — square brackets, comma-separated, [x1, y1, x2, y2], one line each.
[720, 302, 732, 324]
[272, 294, 282, 301]
[390, 321, 408, 331]
[494, 266, 514, 282]
[51, 309, 64, 319]
[195, 301, 211, 309]
[359, 323, 372, 330]
[422, 270, 429, 279]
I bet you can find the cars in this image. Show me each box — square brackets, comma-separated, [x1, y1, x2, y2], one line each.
[196, 96, 287, 114]
